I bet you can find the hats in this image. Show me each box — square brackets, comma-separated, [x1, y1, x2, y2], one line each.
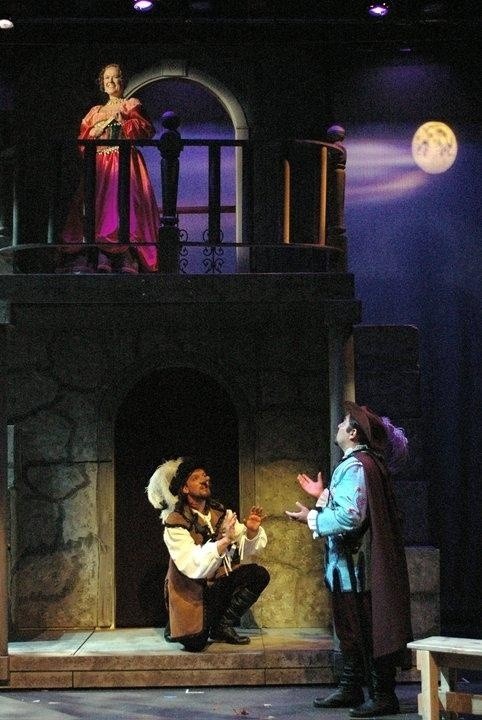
[341, 400, 387, 452]
[169, 454, 208, 496]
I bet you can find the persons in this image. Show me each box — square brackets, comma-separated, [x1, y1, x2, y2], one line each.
[63, 64, 160, 273]
[285, 401, 413, 717]
[163, 461, 270, 652]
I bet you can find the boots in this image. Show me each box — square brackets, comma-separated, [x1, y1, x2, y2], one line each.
[164, 620, 172, 642]
[314, 645, 400, 717]
[210, 585, 259, 644]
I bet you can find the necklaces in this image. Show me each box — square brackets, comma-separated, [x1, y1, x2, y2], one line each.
[107, 98, 126, 105]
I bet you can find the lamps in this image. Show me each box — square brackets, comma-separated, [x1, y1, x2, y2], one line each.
[369, 3, 390, 17]
[0, 14, 15, 28]
[133, 0, 153, 12]
